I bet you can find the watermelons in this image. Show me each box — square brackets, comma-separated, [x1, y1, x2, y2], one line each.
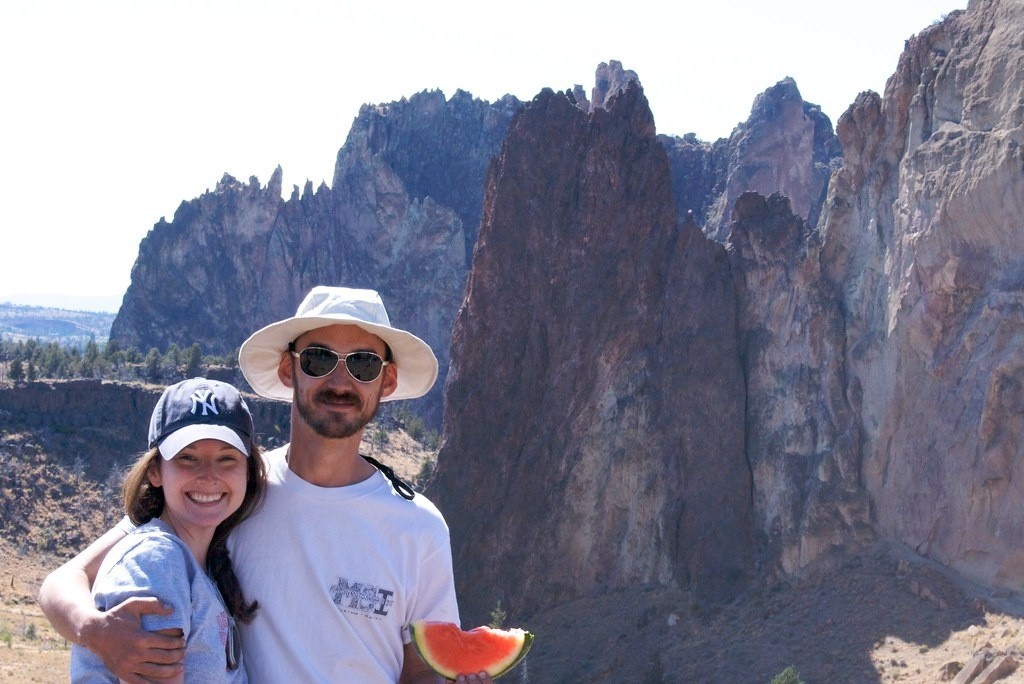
[408, 618, 534, 682]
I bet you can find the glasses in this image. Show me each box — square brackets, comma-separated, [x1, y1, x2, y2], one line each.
[291, 346, 390, 384]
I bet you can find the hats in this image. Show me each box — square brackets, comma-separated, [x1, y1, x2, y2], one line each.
[149, 378, 253, 461]
[239, 286, 439, 403]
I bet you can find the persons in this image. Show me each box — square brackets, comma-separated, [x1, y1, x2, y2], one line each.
[70, 376, 267, 684]
[37, 285, 492, 684]
